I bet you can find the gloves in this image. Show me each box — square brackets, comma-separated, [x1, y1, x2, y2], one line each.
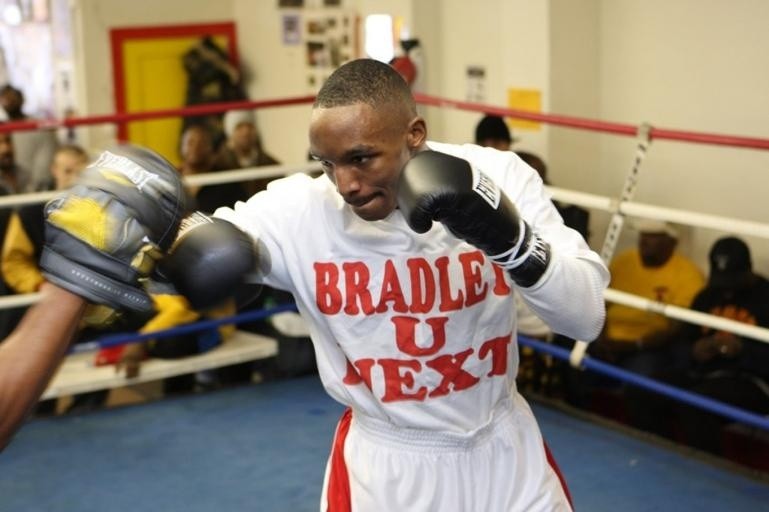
[28, 139, 190, 320]
[144, 209, 261, 314]
[393, 147, 553, 288]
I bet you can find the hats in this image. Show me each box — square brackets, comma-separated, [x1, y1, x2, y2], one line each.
[706, 237, 752, 290]
[626, 217, 687, 242]
[223, 108, 256, 139]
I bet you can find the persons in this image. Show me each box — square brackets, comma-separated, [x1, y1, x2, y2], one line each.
[475, 113, 548, 182]
[605, 220, 769, 453]
[171, 57, 606, 512]
[0, 46, 282, 424]
